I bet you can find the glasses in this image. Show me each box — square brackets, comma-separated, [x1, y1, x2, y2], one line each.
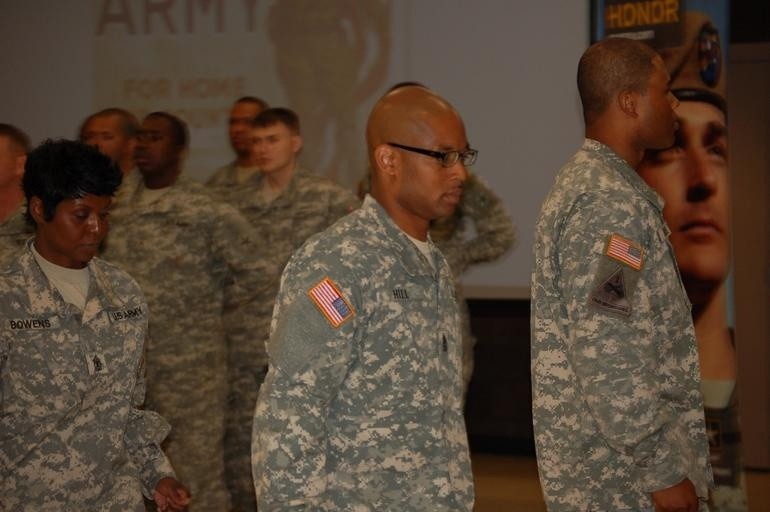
[385, 141, 479, 166]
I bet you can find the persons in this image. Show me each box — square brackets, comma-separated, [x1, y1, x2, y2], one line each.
[0, 124, 31, 225]
[227, 105, 369, 511]
[632, 12, 750, 510]
[83, 112, 268, 510]
[355, 80, 515, 280]
[78, 108, 139, 183]
[287, 0, 391, 192]
[249, 86, 480, 510]
[526, 38, 720, 512]
[1, 140, 191, 512]
[206, 95, 268, 190]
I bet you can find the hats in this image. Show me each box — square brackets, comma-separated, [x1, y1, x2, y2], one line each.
[649, 11, 729, 122]
